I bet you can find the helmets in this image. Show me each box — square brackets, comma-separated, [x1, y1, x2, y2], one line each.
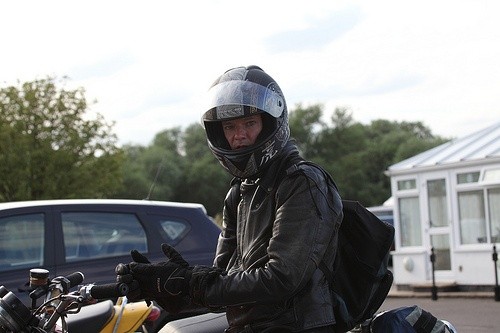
[198, 65, 290, 180]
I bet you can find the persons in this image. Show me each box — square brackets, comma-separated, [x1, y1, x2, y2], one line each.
[113, 65, 396, 333]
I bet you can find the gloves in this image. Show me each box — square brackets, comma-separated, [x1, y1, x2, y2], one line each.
[115, 245, 157, 299]
[126, 241, 209, 307]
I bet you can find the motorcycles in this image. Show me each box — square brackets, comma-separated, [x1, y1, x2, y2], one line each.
[0, 268, 456, 333]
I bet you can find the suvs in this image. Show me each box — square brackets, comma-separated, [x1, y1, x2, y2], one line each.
[0, 198, 225, 309]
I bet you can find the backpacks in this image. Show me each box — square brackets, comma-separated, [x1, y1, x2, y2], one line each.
[230, 152, 394, 333]
